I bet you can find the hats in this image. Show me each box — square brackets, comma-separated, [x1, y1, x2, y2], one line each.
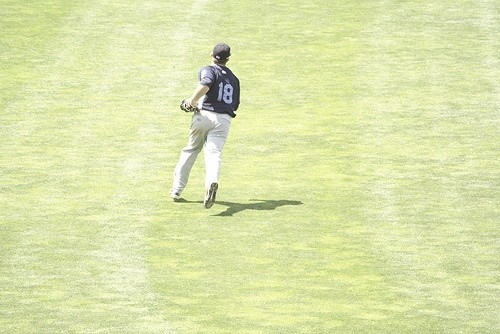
[213, 42, 231, 60]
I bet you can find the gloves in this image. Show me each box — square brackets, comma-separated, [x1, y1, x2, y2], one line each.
[180, 99, 197, 113]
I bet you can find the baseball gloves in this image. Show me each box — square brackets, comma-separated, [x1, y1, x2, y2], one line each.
[180, 99, 199, 114]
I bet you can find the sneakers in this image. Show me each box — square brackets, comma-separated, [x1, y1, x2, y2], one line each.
[204, 182, 218, 209]
[169, 188, 180, 200]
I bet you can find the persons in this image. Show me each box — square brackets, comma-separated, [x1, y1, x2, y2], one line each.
[170, 43, 240, 208]
